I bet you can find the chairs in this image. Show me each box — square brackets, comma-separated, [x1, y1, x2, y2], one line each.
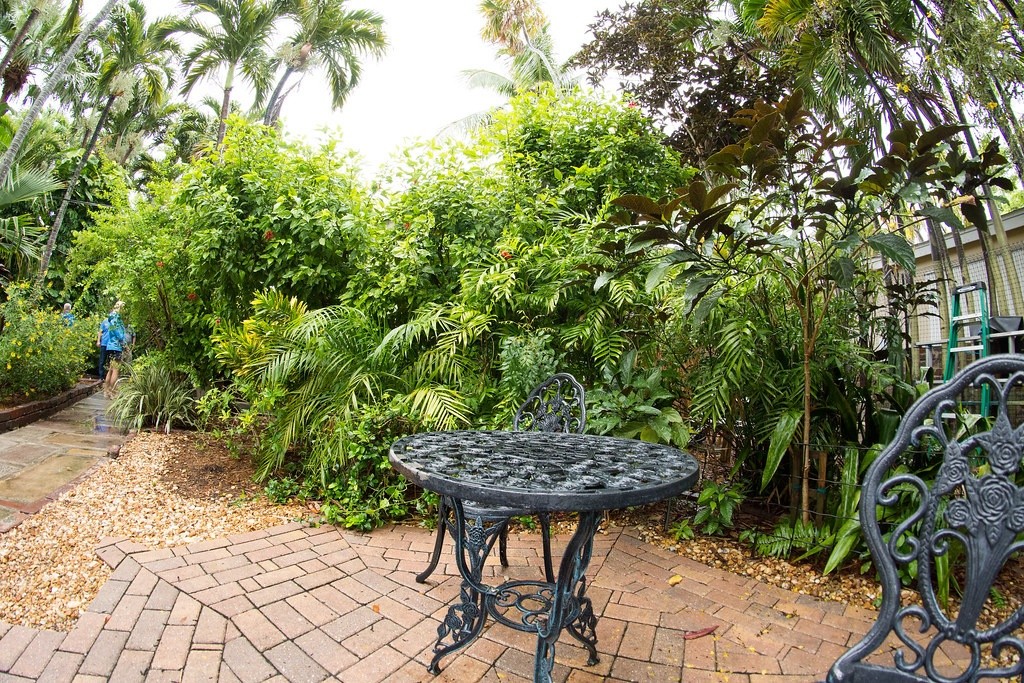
[415, 372, 586, 585]
[826, 353, 1024, 683]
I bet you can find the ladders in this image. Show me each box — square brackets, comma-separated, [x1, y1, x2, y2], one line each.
[926, 280, 994, 474]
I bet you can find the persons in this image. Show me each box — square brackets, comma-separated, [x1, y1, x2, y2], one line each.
[105, 301, 129, 398]
[96, 318, 110, 381]
[62, 303, 75, 325]
[122, 317, 136, 363]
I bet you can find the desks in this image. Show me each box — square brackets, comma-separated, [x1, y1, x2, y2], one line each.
[387, 432, 700, 683]
